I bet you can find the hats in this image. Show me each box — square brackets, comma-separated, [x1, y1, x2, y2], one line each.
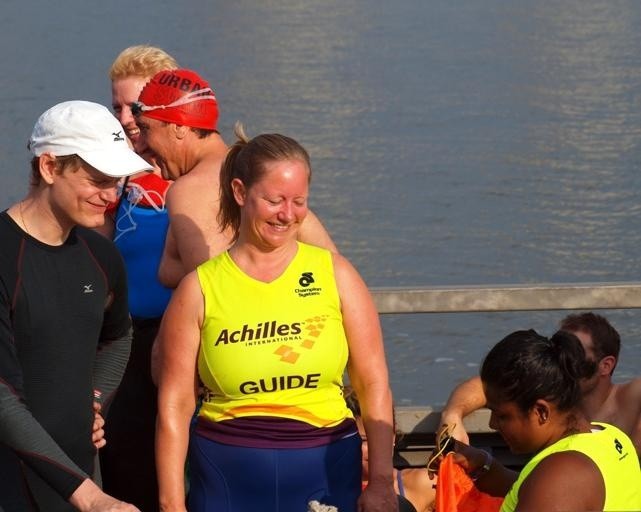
[138, 70, 219, 131]
[29, 100, 154, 178]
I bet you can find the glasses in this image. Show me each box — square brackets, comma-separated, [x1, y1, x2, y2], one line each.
[132, 102, 147, 116]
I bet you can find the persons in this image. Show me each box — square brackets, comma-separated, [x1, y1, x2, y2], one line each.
[1, 99, 142, 511]
[92, 45, 173, 500]
[435, 312, 640, 460]
[428, 329, 641, 512]
[155, 121, 398, 512]
[344, 383, 438, 512]
[132, 70, 340, 402]
